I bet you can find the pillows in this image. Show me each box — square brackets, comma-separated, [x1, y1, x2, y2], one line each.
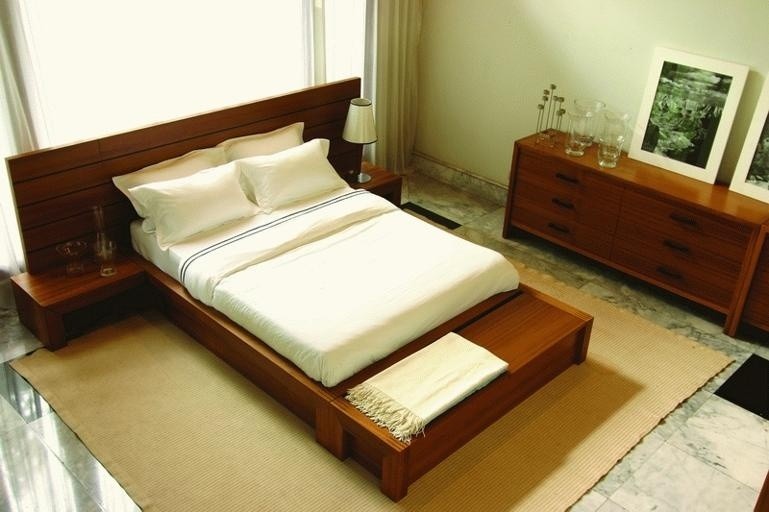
[107, 122, 347, 253]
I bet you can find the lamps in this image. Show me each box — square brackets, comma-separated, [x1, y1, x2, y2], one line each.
[341, 98, 380, 183]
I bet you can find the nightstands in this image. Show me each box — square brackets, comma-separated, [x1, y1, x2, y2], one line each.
[11, 251, 144, 354]
[328, 152, 404, 210]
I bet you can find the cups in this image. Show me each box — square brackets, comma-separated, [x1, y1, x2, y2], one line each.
[56, 238, 95, 286]
[564, 96, 632, 168]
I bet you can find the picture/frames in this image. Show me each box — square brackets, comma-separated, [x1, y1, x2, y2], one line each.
[626, 46, 769, 203]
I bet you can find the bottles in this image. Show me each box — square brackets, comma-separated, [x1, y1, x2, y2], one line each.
[90, 202, 122, 278]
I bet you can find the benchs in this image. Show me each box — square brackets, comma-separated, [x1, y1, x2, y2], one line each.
[331, 293, 596, 504]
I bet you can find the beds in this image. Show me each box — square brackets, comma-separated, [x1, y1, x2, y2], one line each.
[128, 186, 521, 451]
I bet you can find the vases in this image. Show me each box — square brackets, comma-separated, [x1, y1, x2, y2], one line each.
[564, 97, 633, 170]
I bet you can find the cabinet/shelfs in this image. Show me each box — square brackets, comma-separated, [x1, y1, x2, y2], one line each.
[502, 129, 769, 338]
